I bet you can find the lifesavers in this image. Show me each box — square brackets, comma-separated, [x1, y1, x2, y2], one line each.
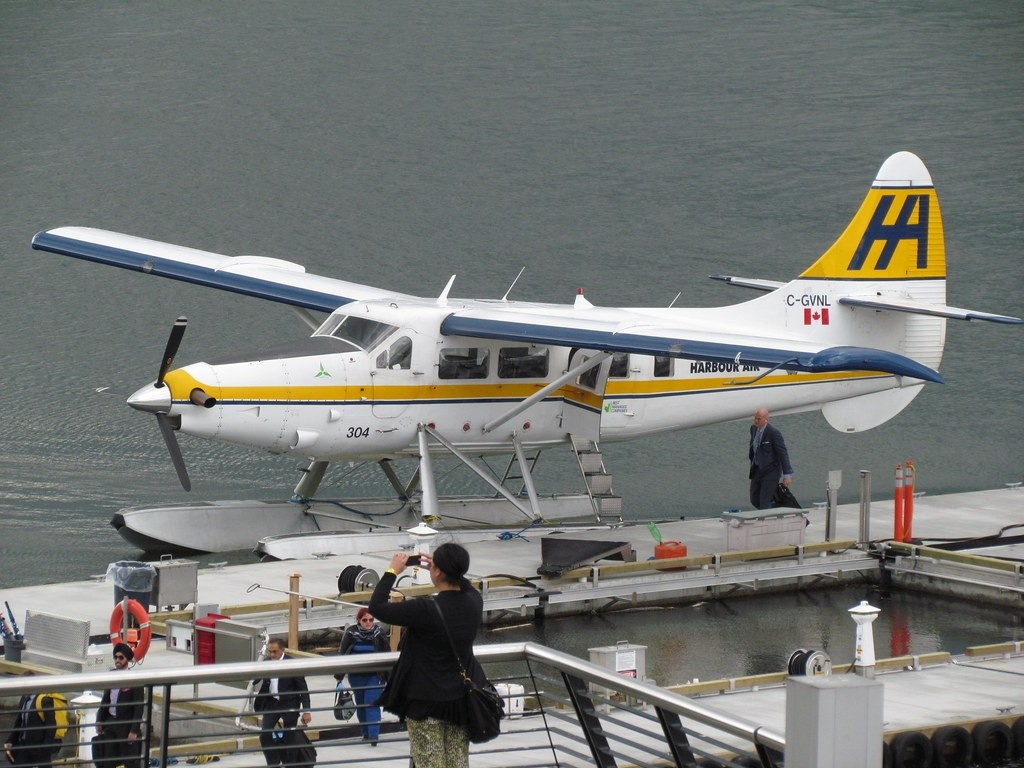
[109, 599, 152, 663]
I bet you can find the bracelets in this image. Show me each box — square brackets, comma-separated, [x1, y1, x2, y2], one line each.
[385, 567, 398, 576]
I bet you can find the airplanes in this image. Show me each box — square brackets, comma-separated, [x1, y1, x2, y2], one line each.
[29, 141, 1024, 563]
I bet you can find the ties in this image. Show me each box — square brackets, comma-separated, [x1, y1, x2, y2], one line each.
[754, 431, 761, 450]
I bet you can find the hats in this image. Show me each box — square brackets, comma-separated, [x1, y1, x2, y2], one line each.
[113, 642, 134, 661]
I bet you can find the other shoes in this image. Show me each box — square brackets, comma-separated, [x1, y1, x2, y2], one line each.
[370, 737, 377, 746]
[362, 735, 368, 741]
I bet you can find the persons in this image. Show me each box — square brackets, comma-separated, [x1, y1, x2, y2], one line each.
[96, 643, 144, 768]
[748, 409, 794, 510]
[368, 542, 483, 768]
[3, 670, 58, 768]
[252, 638, 313, 768]
[334, 607, 392, 747]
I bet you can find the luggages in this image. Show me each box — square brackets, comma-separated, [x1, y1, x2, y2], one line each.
[273, 721, 317, 768]
[774, 483, 809, 528]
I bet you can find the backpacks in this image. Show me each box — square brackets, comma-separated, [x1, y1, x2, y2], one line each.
[36, 692, 69, 739]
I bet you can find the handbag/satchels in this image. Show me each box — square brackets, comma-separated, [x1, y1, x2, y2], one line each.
[464, 675, 507, 743]
[91, 734, 105, 766]
[334, 682, 355, 720]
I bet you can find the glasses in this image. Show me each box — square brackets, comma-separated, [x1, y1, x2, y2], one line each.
[361, 618, 374, 622]
[114, 655, 124, 660]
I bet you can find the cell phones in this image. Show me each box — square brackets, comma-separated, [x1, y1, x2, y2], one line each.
[406, 555, 421, 566]
